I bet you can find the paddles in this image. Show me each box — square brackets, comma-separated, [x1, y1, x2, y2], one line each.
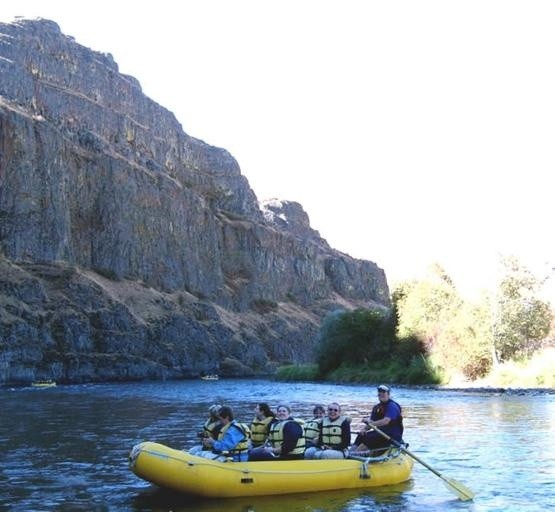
[363, 420, 475, 502]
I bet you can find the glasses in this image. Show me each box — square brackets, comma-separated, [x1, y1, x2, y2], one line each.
[329, 409, 337, 412]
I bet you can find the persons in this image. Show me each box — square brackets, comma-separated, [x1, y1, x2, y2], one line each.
[313, 402, 352, 459]
[248, 404, 307, 460]
[246, 401, 276, 450]
[203, 405, 253, 463]
[189, 403, 222, 458]
[350, 384, 404, 455]
[301, 405, 325, 459]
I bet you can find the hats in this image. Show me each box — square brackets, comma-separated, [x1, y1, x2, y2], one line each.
[377, 383, 391, 393]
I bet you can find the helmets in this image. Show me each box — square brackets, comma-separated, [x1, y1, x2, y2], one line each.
[208, 405, 223, 420]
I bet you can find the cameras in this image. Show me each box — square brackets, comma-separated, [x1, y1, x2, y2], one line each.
[197, 430, 209, 438]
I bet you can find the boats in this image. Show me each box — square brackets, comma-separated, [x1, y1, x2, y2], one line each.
[32, 380, 56, 388]
[129, 438, 414, 497]
[201, 374, 221, 381]
